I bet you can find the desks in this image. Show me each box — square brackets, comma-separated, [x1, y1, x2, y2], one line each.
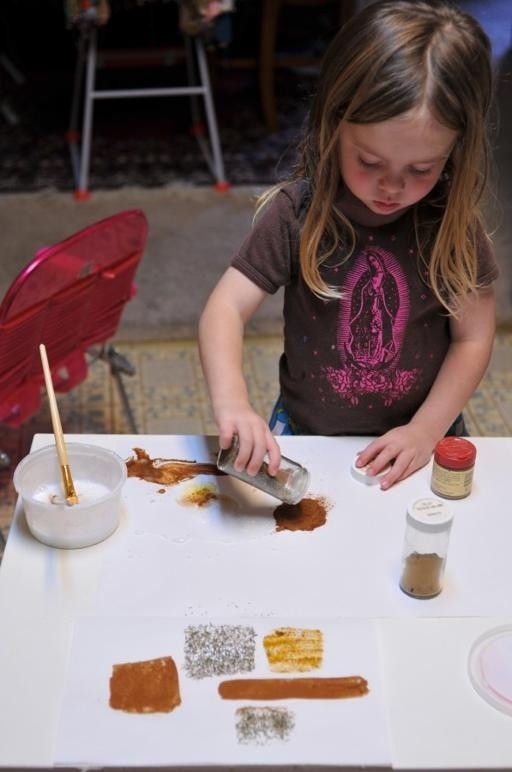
[1, 432, 512, 770]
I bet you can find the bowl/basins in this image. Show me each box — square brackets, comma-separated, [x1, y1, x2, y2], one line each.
[12, 441, 128, 551]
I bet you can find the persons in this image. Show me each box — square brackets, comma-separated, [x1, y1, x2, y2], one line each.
[198, 2, 498, 490]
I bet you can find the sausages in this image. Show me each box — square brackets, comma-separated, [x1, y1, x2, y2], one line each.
[218, 676, 367, 698]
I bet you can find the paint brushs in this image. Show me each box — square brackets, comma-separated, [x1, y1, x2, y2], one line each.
[39, 344, 78, 505]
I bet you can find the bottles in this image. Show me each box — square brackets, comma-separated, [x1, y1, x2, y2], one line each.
[429, 436, 477, 501]
[216, 434, 312, 505]
[398, 496, 455, 600]
